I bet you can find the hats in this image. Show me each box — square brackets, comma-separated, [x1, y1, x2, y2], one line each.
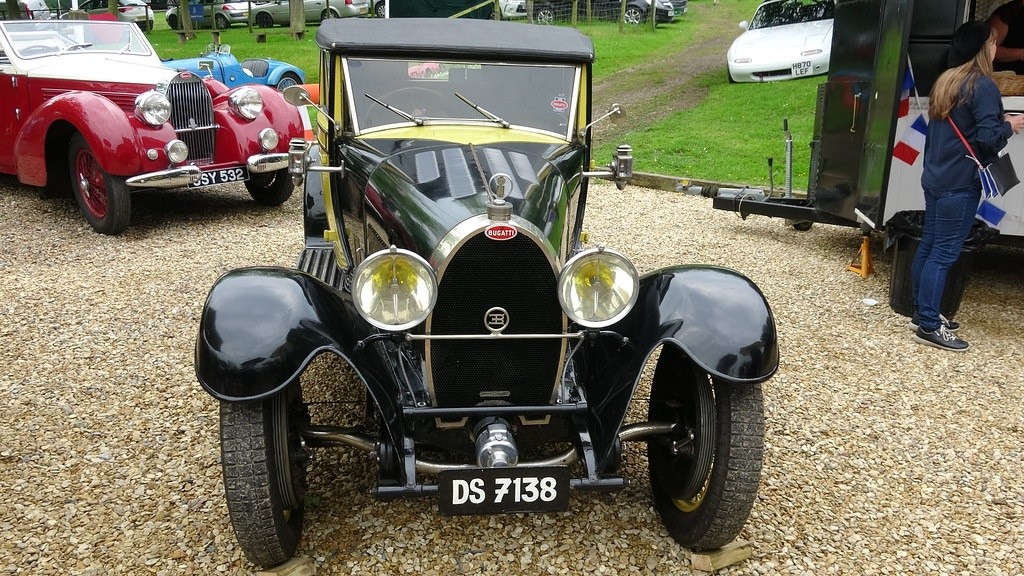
[948, 20, 992, 67]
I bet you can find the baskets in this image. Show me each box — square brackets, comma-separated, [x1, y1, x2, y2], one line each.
[992, 73, 1024, 95]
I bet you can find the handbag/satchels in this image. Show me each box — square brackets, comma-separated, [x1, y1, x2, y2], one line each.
[978, 152, 1020, 199]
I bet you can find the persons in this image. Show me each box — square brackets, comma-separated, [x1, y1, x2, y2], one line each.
[985, 0, 1024, 75]
[909, 18, 1024, 351]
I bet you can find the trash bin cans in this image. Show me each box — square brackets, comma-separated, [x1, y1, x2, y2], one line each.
[889, 211, 988, 320]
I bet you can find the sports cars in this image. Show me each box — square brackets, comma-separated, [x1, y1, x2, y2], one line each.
[727, 0, 840, 84]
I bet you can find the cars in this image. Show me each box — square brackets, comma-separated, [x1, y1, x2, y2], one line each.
[493, 0, 528, 19]
[191, 17, 779, 569]
[160, 43, 306, 92]
[368, 0, 386, 18]
[250, 0, 370, 28]
[0, 8, 309, 239]
[0, 1, 52, 21]
[530, 0, 675, 26]
[165, 0, 258, 30]
[59, 0, 154, 31]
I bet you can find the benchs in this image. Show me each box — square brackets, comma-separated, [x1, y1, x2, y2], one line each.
[251, 30, 309, 43]
[171, 30, 227, 45]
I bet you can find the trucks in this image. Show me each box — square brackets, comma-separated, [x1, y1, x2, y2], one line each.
[693, 0, 1024, 261]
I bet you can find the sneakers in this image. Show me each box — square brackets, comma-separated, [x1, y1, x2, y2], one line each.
[914, 324, 970, 351]
[909, 310, 960, 332]
